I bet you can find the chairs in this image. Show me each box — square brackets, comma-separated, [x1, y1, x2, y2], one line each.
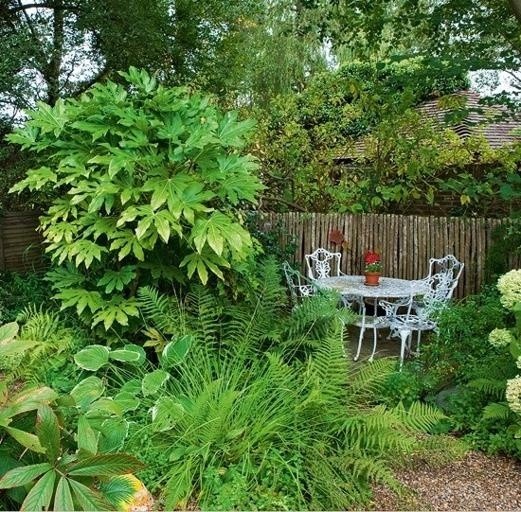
[368, 272, 454, 374]
[282, 260, 348, 359]
[388, 254, 466, 357]
[304, 248, 363, 326]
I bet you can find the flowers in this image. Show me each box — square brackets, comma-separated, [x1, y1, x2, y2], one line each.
[364, 251, 382, 272]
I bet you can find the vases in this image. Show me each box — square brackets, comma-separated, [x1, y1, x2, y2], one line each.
[364, 272, 381, 286]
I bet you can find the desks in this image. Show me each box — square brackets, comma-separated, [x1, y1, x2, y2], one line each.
[312, 275, 428, 362]
